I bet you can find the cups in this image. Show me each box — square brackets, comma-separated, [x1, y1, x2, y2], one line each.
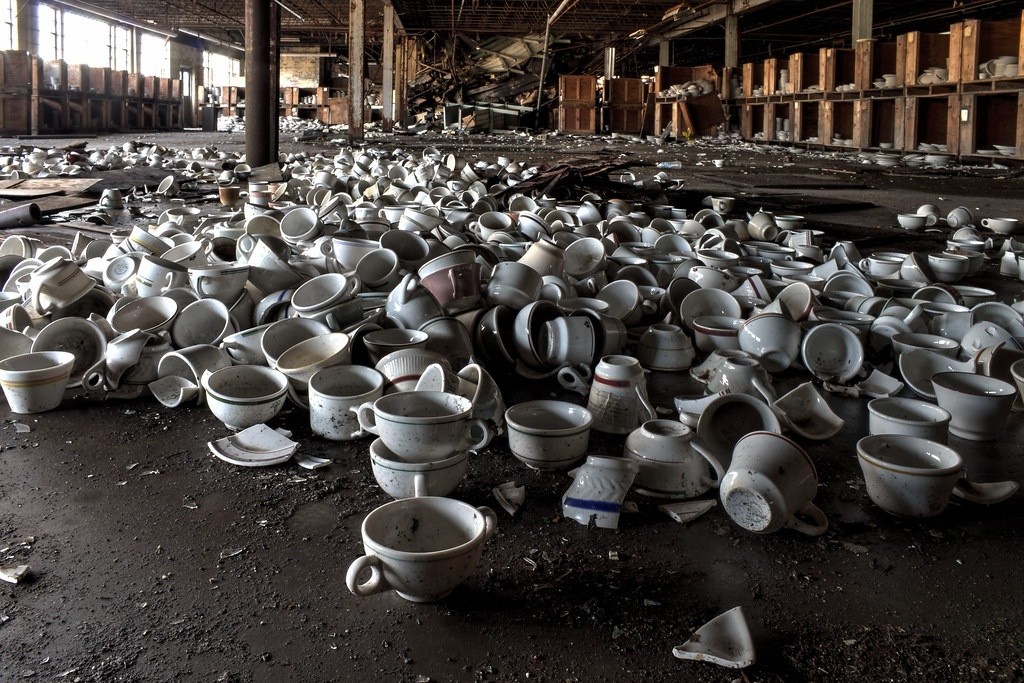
[0, 55, 1023, 605]
